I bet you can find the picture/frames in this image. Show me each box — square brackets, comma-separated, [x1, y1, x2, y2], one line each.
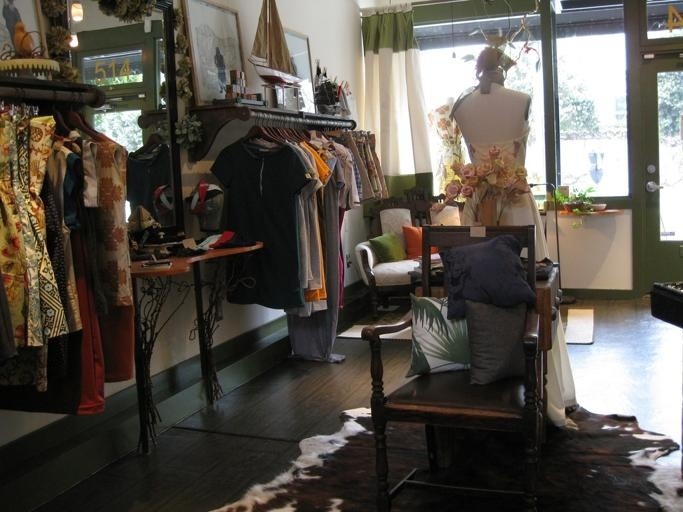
[181, 0, 249, 107]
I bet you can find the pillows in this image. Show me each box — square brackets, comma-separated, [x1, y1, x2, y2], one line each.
[369, 231, 407, 262]
[405, 234, 537, 385]
[403, 227, 439, 259]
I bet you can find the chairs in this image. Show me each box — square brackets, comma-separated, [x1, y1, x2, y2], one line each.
[361, 225, 540, 512]
[355, 173, 465, 318]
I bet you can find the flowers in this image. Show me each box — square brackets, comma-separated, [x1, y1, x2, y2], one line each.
[430, 147, 531, 214]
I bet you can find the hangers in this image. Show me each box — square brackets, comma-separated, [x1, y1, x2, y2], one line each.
[17, 87, 98, 139]
[243, 112, 346, 151]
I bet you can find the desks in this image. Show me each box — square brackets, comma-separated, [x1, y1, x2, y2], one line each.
[408, 262, 563, 471]
[131, 241, 264, 455]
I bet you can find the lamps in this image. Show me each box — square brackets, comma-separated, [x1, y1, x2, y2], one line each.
[69, 32, 79, 48]
[71, 0, 83, 21]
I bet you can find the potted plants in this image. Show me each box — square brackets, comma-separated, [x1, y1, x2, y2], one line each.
[553, 187, 595, 228]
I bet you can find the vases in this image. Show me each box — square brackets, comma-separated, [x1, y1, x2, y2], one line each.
[478, 199, 497, 226]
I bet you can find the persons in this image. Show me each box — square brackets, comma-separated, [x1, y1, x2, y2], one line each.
[445, 44, 583, 435]
[212, 46, 229, 93]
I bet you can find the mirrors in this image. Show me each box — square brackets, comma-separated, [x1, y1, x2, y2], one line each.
[62, 0, 185, 243]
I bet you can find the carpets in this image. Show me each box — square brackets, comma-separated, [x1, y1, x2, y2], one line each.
[208, 407, 683, 512]
[336, 302, 594, 344]
